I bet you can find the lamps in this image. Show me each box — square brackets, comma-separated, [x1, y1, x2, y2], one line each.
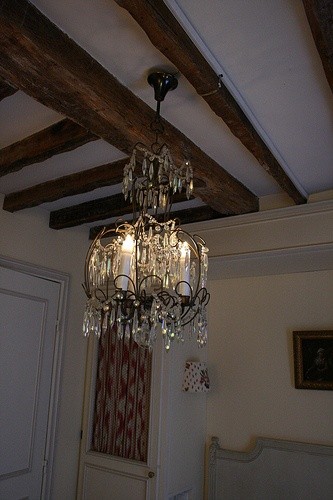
[82, 72, 211, 353]
[182, 361, 211, 394]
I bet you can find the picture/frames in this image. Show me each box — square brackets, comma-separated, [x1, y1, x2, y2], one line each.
[292, 330, 333, 391]
